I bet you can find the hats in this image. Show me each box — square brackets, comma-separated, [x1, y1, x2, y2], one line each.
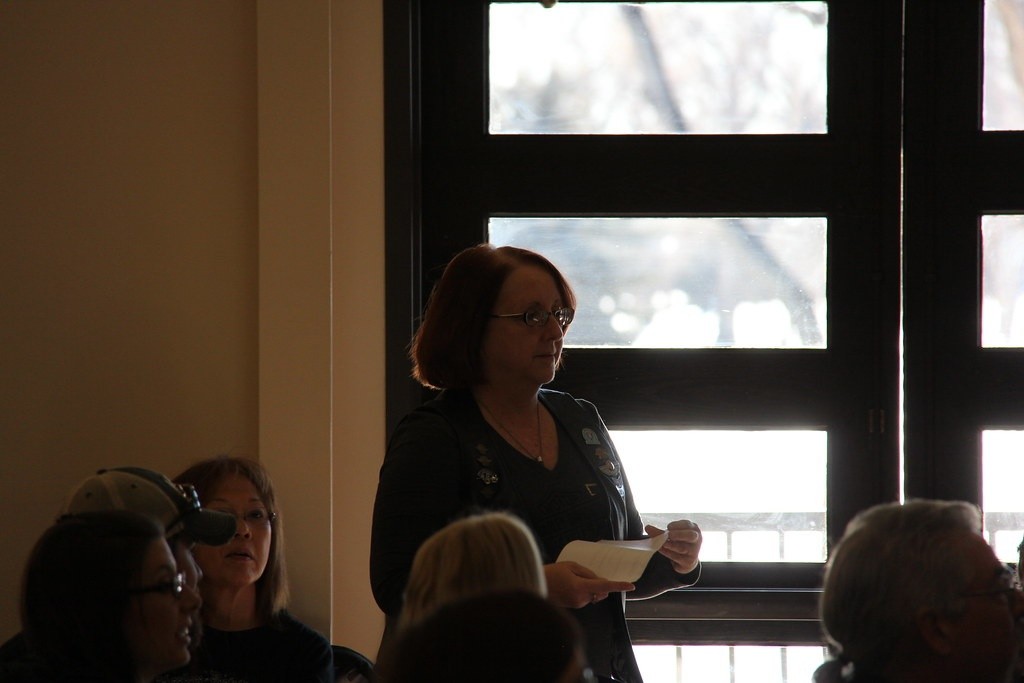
[68, 466, 239, 547]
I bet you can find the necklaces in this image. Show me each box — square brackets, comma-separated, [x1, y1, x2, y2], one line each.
[477, 395, 545, 462]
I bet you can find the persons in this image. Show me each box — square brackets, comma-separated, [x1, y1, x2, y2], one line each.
[368, 242, 704, 683]
[2, 454, 1023, 683]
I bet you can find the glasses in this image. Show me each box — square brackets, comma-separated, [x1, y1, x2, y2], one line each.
[485, 305, 575, 328]
[936, 561, 1024, 603]
[116, 569, 187, 601]
[212, 506, 276, 527]
